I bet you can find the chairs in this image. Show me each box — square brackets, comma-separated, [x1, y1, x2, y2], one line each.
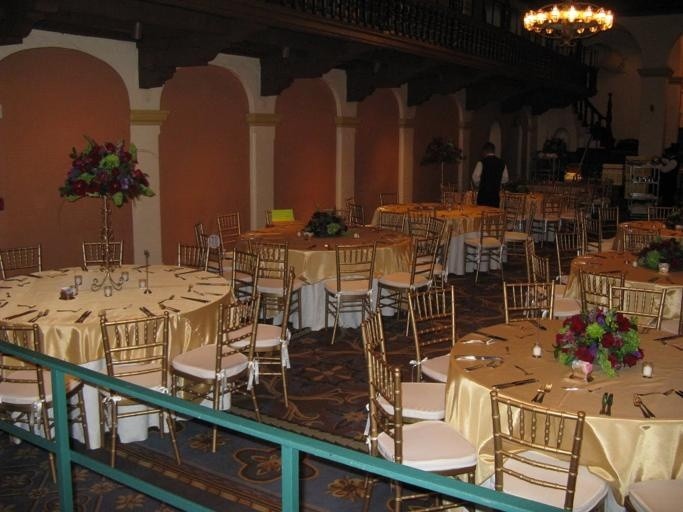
[82, 240, 123, 266]
[96, 310, 182, 469]
[0, 324, 91, 484]
[194, 172, 682, 346]
[177, 242, 210, 271]
[495, 170, 683, 512]
[0, 244, 41, 280]
[361, 308, 447, 431]
[168, 290, 263, 453]
[228, 266, 297, 408]
[408, 285, 456, 382]
[363, 343, 477, 512]
[624, 480, 683, 512]
[488, 390, 610, 511]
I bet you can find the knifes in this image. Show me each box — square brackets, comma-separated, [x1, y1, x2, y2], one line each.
[179, 296, 209, 303]
[492, 378, 537, 391]
[472, 330, 508, 342]
[4, 308, 36, 320]
[139, 305, 157, 317]
[599, 392, 614, 417]
[675, 390, 682, 398]
[74, 310, 91, 324]
[530, 318, 547, 331]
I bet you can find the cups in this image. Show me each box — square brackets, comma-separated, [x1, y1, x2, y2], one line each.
[103, 285, 113, 298]
[73, 274, 82, 286]
[121, 271, 129, 281]
[642, 361, 653, 378]
[532, 342, 542, 358]
[658, 263, 669, 273]
[139, 279, 145, 288]
[631, 259, 638, 268]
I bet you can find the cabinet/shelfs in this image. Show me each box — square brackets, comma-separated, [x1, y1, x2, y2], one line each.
[624, 155, 661, 215]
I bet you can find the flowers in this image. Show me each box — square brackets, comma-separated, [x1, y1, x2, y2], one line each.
[59, 133, 157, 208]
[421, 135, 467, 166]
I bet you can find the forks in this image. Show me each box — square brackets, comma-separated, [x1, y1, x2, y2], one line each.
[632, 393, 656, 419]
[463, 359, 506, 373]
[56, 307, 82, 314]
[530, 380, 553, 403]
[157, 294, 175, 305]
[29, 309, 50, 323]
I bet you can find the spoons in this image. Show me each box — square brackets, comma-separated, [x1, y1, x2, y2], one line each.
[16, 303, 36, 308]
[637, 387, 677, 396]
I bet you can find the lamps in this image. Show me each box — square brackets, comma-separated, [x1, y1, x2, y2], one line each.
[522, 0, 615, 46]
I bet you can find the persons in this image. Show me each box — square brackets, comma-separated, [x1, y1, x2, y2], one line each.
[472, 142, 510, 208]
[542, 127, 571, 182]
